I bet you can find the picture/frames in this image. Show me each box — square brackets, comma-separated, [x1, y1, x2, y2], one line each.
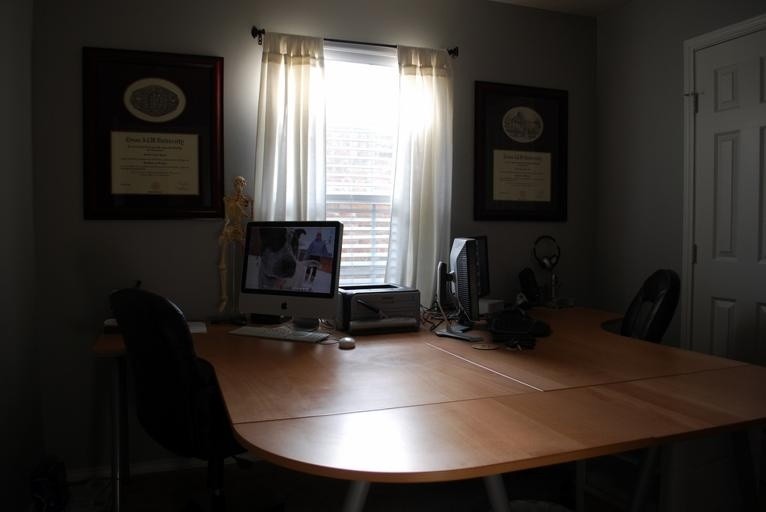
[473, 80, 568, 223]
[82, 44, 223, 221]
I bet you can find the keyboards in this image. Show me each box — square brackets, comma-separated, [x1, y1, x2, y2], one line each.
[492, 318, 528, 335]
[228, 325, 330, 344]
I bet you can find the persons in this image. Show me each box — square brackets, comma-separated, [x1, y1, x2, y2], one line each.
[258, 227, 329, 290]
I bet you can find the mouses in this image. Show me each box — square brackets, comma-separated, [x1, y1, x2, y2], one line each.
[339, 336, 355, 349]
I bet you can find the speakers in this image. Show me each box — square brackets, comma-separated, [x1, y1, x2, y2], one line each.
[519, 267, 541, 302]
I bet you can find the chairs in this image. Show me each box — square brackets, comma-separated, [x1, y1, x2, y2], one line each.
[621, 269, 680, 343]
[109, 286, 249, 510]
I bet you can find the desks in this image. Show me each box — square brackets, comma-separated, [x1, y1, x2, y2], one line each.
[190, 292, 766, 510]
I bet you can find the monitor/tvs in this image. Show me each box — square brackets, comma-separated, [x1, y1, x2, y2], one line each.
[434, 235, 490, 342]
[239, 221, 343, 332]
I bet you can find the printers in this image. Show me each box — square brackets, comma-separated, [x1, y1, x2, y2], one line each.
[325, 285, 422, 333]
